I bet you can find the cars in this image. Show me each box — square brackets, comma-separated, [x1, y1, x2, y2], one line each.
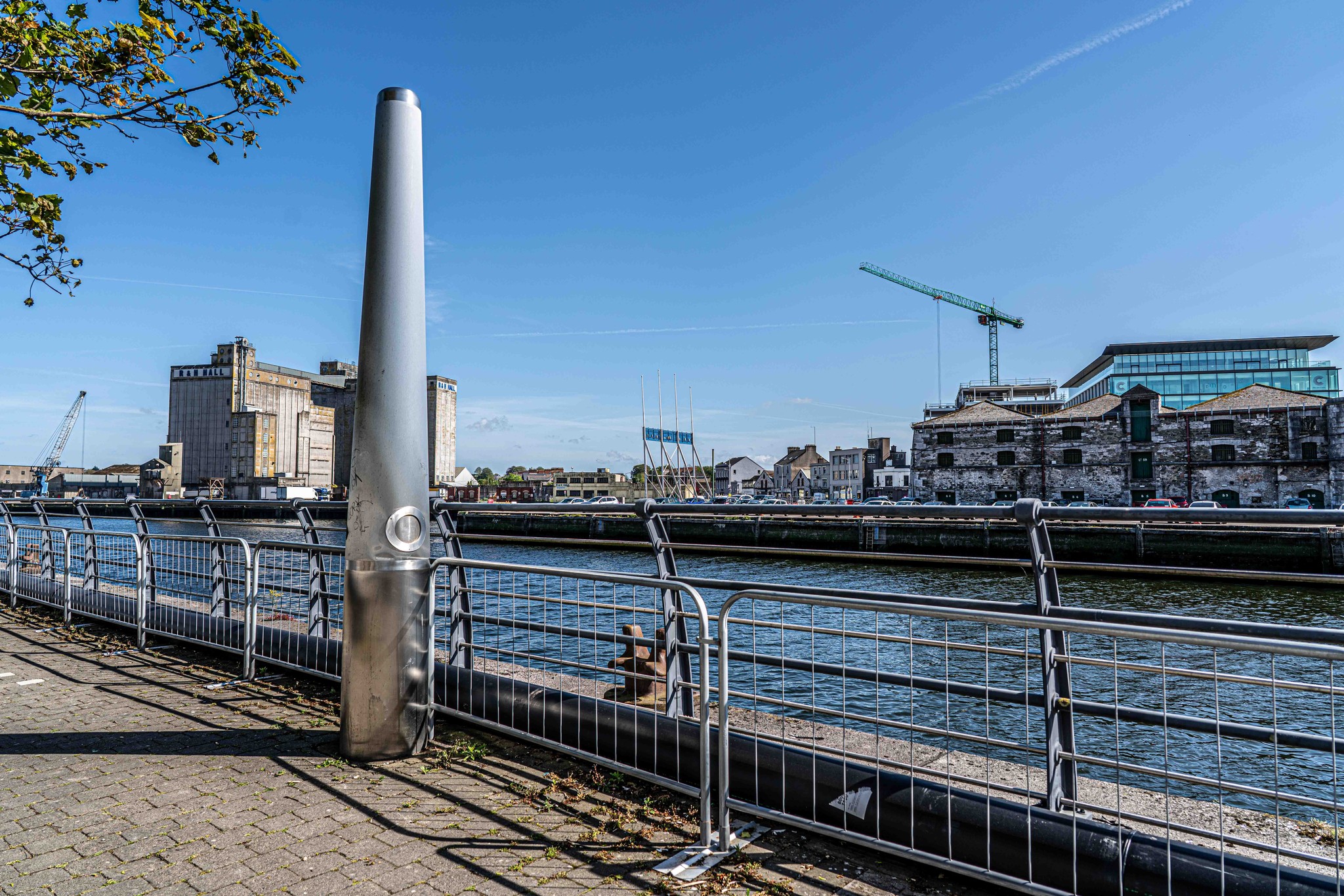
[731, 495, 790, 516]
[653, 497, 720, 504]
[557, 497, 588, 504]
[801, 495, 1017, 521]
[1140, 495, 1228, 524]
[1040, 497, 1112, 523]
[1281, 498, 1316, 510]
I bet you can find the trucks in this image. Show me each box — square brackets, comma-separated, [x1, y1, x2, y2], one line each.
[261, 486, 330, 502]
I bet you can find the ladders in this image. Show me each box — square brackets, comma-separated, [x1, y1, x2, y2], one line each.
[865, 520, 874, 551]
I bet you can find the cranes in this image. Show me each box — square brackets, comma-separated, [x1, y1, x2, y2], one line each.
[858, 261, 1026, 384]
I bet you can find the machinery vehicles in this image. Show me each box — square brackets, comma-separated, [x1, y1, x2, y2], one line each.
[19, 390, 90, 498]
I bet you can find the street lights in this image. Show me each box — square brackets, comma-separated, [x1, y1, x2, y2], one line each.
[625, 472, 631, 482]
[681, 473, 688, 484]
[775, 464, 783, 498]
[723, 468, 731, 484]
[840, 457, 851, 499]
[634, 476, 639, 484]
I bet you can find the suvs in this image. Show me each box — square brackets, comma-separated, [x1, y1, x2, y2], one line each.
[581, 496, 620, 504]
[712, 494, 740, 515]
